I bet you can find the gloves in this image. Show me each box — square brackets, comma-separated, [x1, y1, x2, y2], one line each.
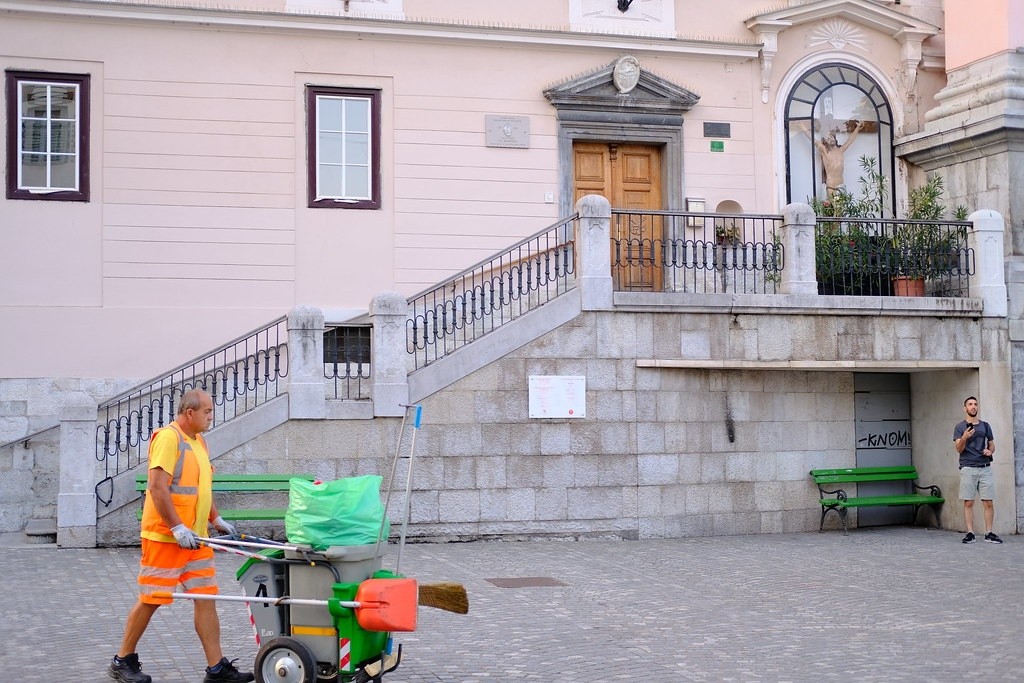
[212, 516, 239, 538]
[170, 524, 200, 550]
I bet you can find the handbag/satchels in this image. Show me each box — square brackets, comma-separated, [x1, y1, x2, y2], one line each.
[984, 422, 993, 462]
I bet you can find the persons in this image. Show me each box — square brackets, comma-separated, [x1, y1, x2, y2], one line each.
[107, 390, 255, 683]
[794, 118, 865, 233]
[953, 397, 1004, 544]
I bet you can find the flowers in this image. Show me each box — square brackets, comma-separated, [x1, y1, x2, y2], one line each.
[762, 154, 968, 288]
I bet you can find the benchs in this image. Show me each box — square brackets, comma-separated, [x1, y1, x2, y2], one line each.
[809, 465, 945, 535]
[134, 474, 314, 521]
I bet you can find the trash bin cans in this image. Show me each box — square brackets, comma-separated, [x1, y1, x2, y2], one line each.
[235, 544, 289, 650]
[281, 542, 384, 668]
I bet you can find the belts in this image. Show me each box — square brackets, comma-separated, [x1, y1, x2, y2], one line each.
[977, 464, 990, 467]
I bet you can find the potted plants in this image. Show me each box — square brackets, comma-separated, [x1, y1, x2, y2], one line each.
[716, 223, 739, 245]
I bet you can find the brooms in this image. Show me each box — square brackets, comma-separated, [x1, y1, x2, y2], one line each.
[418, 583, 469, 614]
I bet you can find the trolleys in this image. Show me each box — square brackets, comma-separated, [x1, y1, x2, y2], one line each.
[191, 533, 403, 683]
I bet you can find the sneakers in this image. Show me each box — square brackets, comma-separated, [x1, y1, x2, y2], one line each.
[962, 532, 976, 543]
[204, 657, 254, 683]
[984, 532, 1003, 544]
[107, 653, 152, 683]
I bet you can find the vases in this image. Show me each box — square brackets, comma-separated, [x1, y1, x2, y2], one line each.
[890, 276, 927, 297]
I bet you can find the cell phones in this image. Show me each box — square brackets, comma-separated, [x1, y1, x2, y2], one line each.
[966, 423, 973, 431]
